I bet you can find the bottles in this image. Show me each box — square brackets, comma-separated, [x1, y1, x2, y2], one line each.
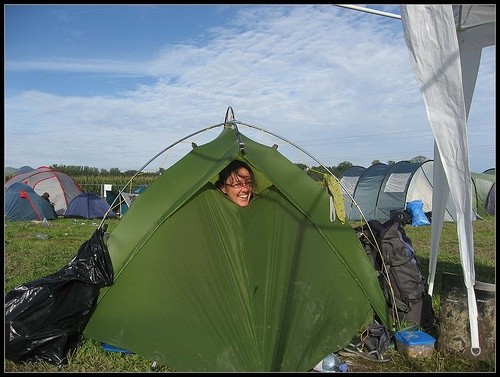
[322, 354, 347, 372]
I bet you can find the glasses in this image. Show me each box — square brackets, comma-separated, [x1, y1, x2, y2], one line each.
[224, 181, 252, 189]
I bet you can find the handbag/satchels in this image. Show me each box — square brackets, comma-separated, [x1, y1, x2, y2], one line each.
[389, 200, 429, 225]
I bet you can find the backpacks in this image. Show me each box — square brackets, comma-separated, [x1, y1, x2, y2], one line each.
[354, 216, 438, 333]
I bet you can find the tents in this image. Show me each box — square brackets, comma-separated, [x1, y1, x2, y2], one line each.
[4, 166, 115, 221]
[83, 128, 390, 372]
[477, 168, 496, 214]
[332, 160, 478, 224]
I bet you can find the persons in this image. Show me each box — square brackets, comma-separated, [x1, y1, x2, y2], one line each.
[215, 159, 255, 206]
